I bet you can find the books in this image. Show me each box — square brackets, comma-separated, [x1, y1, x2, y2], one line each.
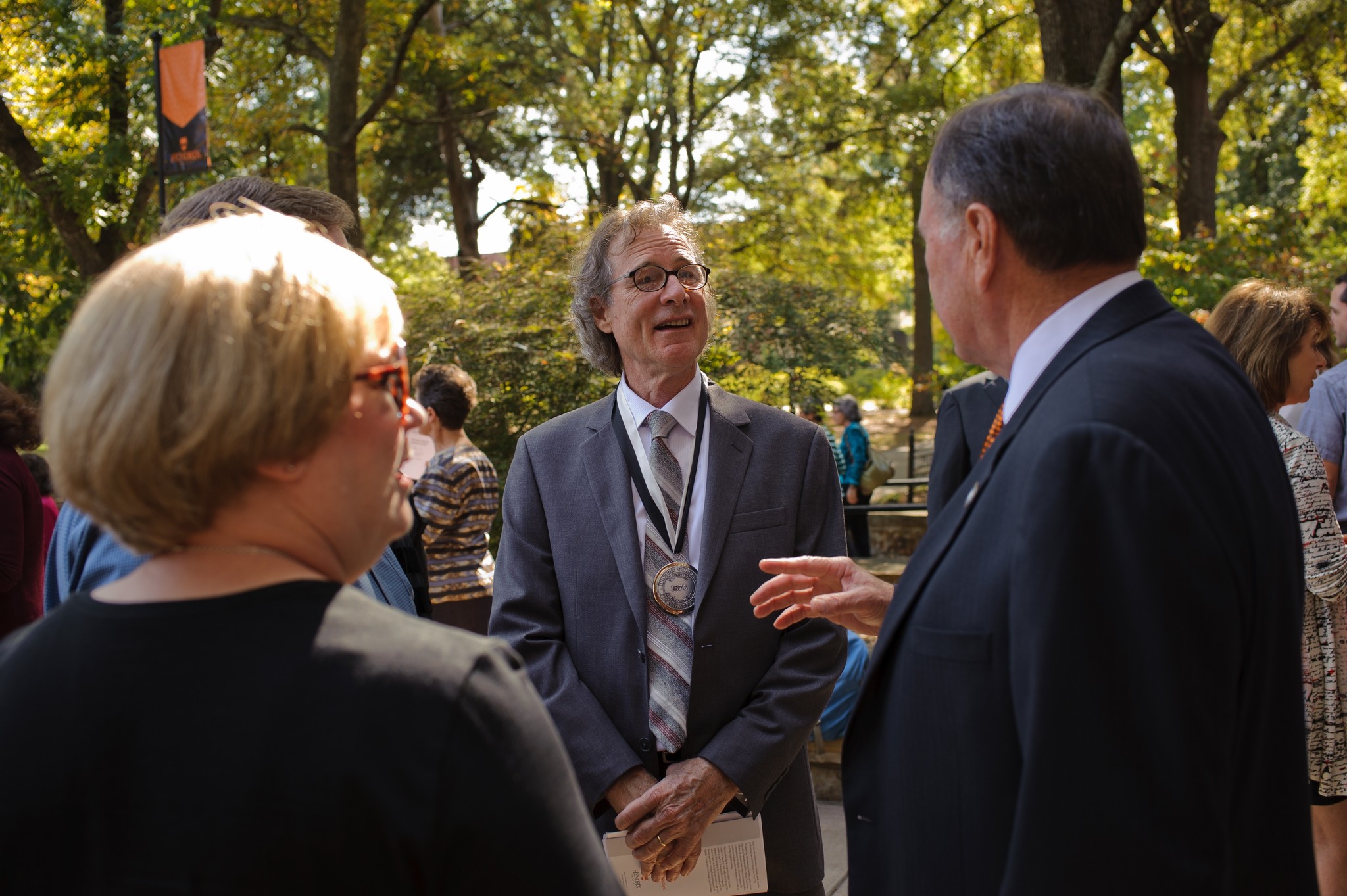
[603, 810, 768, 896]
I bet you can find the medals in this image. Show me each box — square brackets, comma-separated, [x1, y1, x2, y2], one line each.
[653, 562, 698, 614]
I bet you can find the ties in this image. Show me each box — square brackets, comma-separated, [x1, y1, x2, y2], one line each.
[641, 410, 693, 753]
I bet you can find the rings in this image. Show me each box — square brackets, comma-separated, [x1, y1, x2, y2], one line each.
[656, 835, 666, 847]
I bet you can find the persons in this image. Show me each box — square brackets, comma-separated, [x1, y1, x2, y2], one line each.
[0, 215, 619, 895]
[835, 397, 872, 560]
[799, 397, 845, 499]
[21, 454, 58, 620]
[486, 196, 848, 892]
[751, 82, 1321, 895]
[1299, 274, 1347, 542]
[926, 368, 1010, 530]
[1198, 275, 1347, 894]
[409, 364, 497, 638]
[0, 383, 41, 638]
[44, 180, 419, 622]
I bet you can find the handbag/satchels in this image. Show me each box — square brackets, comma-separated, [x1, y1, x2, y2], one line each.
[858, 445, 895, 495]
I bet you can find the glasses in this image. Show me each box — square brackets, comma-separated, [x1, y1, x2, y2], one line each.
[353, 335, 410, 417]
[607, 263, 711, 292]
